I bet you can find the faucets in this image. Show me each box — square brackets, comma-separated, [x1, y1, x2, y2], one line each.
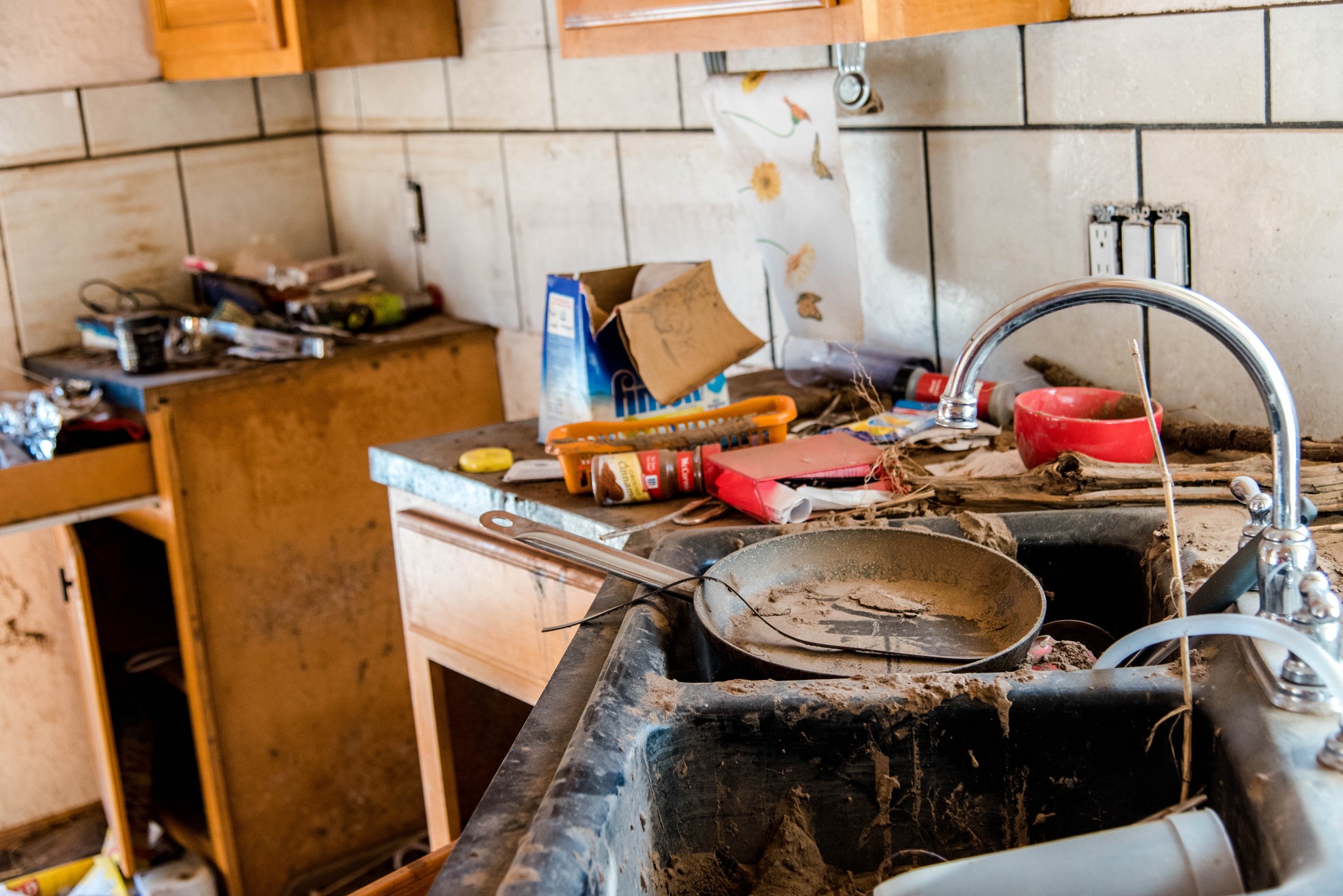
[938, 274, 1343, 717]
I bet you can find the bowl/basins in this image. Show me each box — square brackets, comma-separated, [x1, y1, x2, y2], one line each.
[1014, 386, 1163, 473]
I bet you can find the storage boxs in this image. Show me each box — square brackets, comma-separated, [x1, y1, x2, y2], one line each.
[536, 260, 768, 448]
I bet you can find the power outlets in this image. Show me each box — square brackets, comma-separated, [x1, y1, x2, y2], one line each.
[1084, 200, 1191, 290]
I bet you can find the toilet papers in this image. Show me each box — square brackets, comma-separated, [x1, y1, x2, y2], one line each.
[700, 67, 867, 345]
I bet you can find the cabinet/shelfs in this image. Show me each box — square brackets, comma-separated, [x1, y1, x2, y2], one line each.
[0, 286, 505, 896]
[357, 364, 1343, 854]
[138, 0, 465, 88]
[546, 0, 1075, 64]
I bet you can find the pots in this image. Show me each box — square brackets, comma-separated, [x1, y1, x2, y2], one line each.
[480, 510, 1046, 679]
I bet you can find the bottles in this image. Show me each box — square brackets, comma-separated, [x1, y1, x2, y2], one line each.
[344, 286, 441, 328]
[782, 336, 1016, 428]
[590, 442, 722, 508]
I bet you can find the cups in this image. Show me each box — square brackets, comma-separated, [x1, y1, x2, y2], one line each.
[874, 807, 1244, 896]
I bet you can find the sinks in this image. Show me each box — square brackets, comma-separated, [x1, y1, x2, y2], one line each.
[429, 678, 1343, 896]
[593, 506, 1218, 718]
